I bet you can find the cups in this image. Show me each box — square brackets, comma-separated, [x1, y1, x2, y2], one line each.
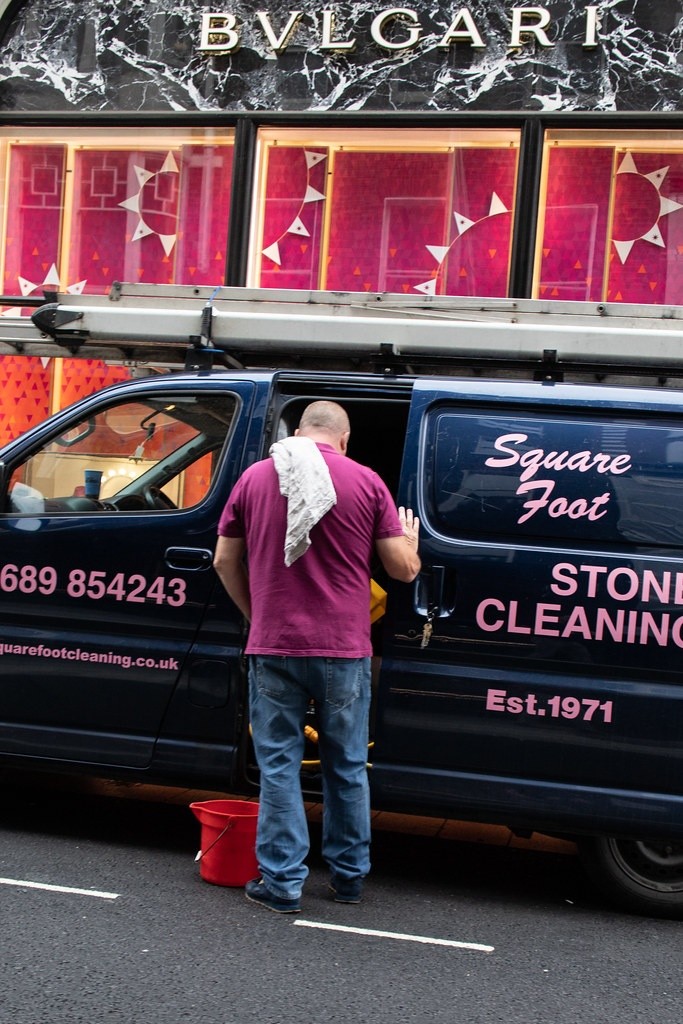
[84, 470, 103, 500]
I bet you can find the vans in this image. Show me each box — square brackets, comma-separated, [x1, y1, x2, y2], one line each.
[0, 368, 683, 922]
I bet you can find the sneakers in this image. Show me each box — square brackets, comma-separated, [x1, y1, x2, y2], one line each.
[329, 875, 364, 903]
[244, 877, 302, 912]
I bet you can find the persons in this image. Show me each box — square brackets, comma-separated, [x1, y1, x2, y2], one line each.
[213, 401, 419, 915]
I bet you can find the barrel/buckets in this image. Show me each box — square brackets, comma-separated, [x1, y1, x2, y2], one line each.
[187, 798, 263, 888]
